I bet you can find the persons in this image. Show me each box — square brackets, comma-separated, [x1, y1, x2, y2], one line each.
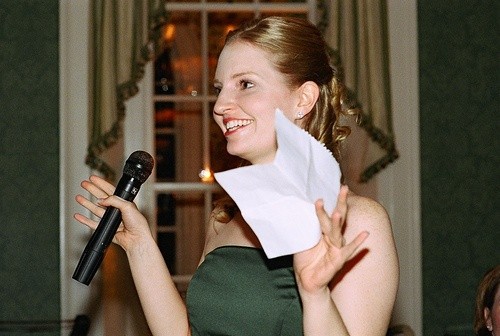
[476, 266, 500, 336]
[73, 14, 398, 336]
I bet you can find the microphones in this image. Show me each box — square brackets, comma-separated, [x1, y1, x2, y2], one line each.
[72, 151, 155, 286]
